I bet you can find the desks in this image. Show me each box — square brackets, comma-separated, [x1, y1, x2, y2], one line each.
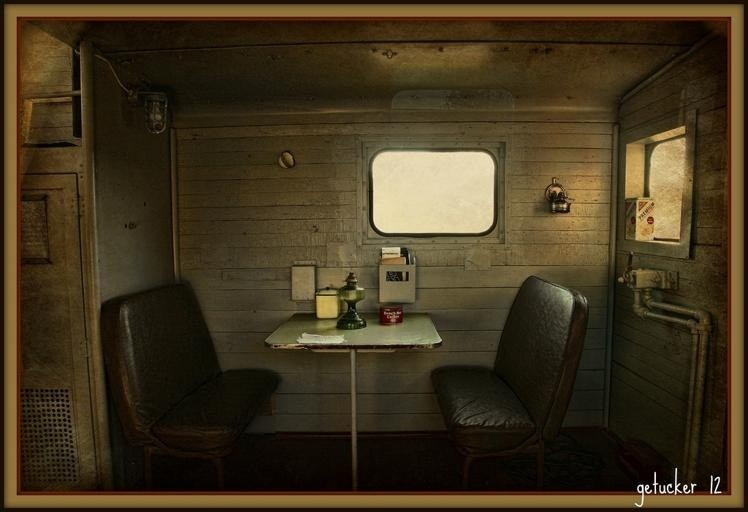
[265, 312, 442, 489]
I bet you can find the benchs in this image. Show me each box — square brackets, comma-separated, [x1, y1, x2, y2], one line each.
[101, 278, 282, 491]
[432, 274, 590, 492]
